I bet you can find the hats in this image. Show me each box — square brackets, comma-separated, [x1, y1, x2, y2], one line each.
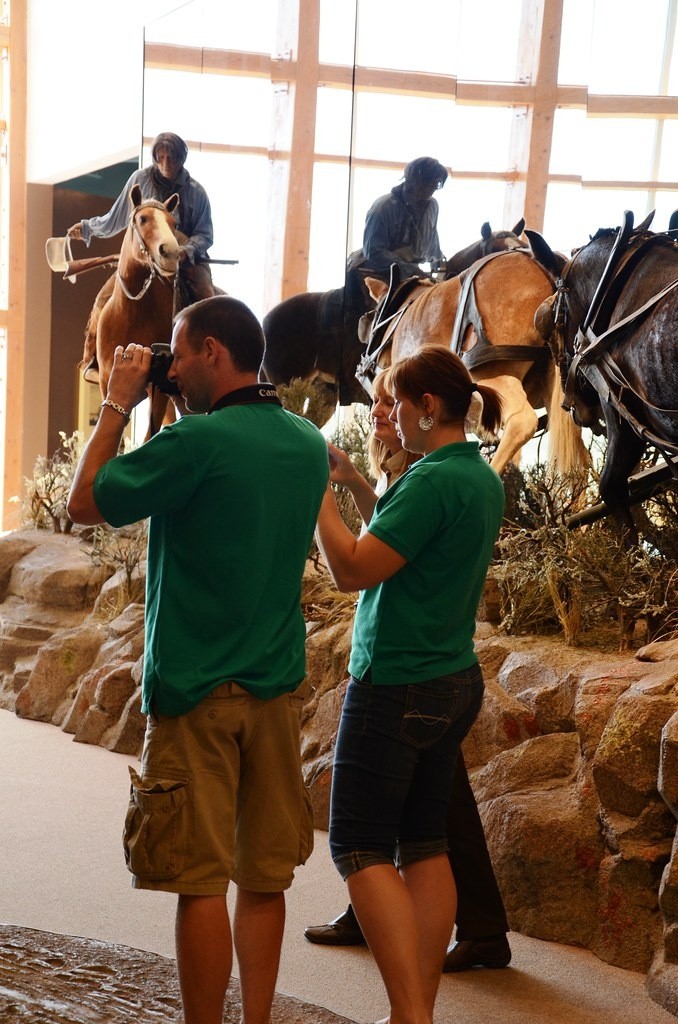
[45, 231, 77, 284]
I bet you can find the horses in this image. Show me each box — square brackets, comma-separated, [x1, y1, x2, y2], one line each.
[260, 216, 533, 429]
[538, 209, 678, 567]
[78, 182, 228, 542]
[358, 249, 591, 498]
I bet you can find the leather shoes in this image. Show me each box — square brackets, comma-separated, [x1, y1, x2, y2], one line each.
[304, 911, 369, 945]
[438, 932, 514, 974]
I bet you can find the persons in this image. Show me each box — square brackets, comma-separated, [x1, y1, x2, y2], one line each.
[358, 155, 450, 308]
[305, 358, 512, 975]
[317, 344, 505, 1024]
[63, 132, 219, 302]
[66, 295, 330, 1024]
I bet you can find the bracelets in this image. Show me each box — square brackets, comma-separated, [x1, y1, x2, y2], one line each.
[100, 399, 131, 422]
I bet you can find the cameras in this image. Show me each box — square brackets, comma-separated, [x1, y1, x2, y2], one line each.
[121, 343, 184, 396]
